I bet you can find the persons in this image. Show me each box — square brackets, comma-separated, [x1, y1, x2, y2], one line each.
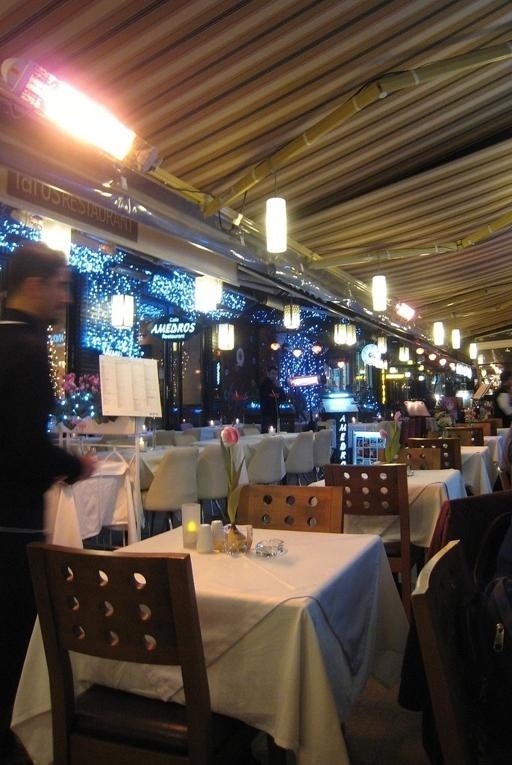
[436, 382, 459, 421]
[0, 241, 97, 765]
[493, 370, 512, 428]
[254, 366, 287, 433]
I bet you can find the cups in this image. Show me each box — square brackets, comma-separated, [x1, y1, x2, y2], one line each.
[196, 519, 223, 552]
[181, 502, 202, 548]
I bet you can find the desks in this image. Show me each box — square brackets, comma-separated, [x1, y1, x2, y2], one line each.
[11, 524, 408, 765]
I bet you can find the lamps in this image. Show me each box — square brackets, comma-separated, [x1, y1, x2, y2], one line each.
[0, 54, 163, 175]
[110, 196, 501, 382]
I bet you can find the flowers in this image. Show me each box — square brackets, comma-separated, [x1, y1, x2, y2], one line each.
[478, 408, 491, 421]
[464, 408, 476, 426]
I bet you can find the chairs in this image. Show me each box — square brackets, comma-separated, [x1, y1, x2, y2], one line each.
[411, 539, 481, 764]
[29, 540, 285, 765]
[236, 484, 344, 536]
[323, 463, 412, 620]
[27, 418, 510, 547]
